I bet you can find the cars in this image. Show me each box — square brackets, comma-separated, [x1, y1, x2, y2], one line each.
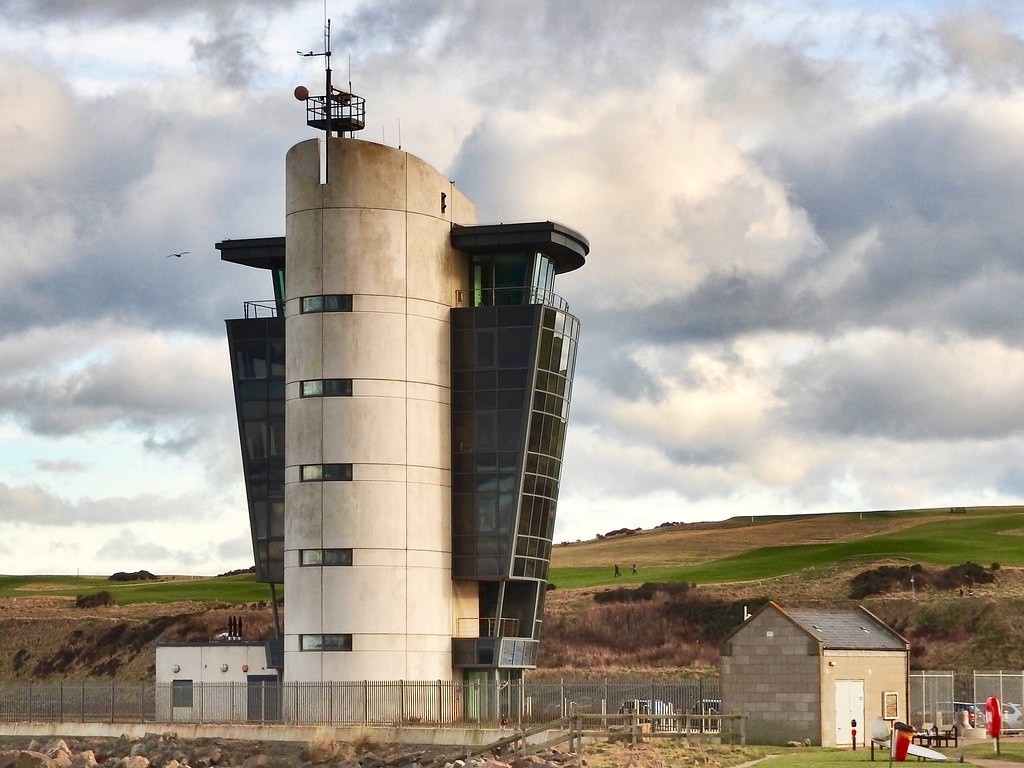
[972, 702, 1024, 735]
[910, 701, 985, 735]
[684, 699, 721, 730]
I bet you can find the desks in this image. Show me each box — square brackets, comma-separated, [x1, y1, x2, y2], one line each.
[911, 726, 958, 750]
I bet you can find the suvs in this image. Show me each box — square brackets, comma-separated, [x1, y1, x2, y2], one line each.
[615, 699, 677, 728]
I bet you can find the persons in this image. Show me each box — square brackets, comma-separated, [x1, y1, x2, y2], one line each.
[959, 585, 974, 598]
[615, 564, 620, 577]
[633, 563, 637, 574]
[705, 708, 718, 729]
[500, 714, 506, 727]
[641, 702, 652, 723]
